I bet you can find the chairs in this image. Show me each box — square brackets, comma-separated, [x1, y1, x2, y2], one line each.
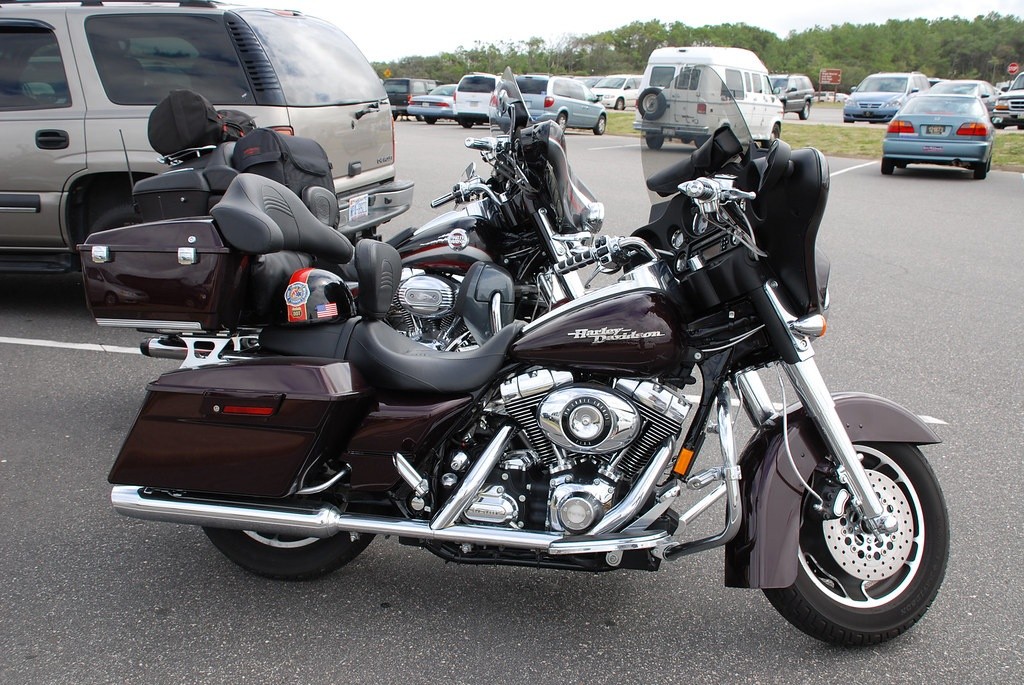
[158, 65, 190, 97]
[120, 58, 145, 101]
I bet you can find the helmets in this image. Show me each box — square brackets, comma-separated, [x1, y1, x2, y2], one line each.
[274, 266, 358, 328]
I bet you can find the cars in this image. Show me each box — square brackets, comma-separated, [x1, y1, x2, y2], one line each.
[770, 74, 815, 120]
[490, 75, 607, 136]
[993, 72, 1024, 129]
[452, 74, 501, 128]
[590, 75, 642, 110]
[20, 43, 69, 84]
[383, 78, 443, 121]
[900, 80, 1004, 112]
[880, 95, 996, 180]
[406, 84, 458, 124]
[813, 91, 849, 103]
[574, 76, 603, 89]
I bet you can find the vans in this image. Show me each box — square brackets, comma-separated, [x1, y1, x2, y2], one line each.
[633, 47, 784, 149]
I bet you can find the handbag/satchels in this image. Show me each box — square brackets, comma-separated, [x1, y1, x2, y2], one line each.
[147, 88, 257, 161]
[232, 127, 336, 201]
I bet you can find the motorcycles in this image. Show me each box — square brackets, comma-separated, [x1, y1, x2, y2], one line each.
[75, 67, 605, 369]
[107, 64, 949, 647]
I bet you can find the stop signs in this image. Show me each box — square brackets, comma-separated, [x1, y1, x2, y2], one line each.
[1008, 63, 1018, 74]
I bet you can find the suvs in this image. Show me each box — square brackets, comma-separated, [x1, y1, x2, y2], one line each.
[0, 0, 414, 278]
[843, 72, 931, 124]
